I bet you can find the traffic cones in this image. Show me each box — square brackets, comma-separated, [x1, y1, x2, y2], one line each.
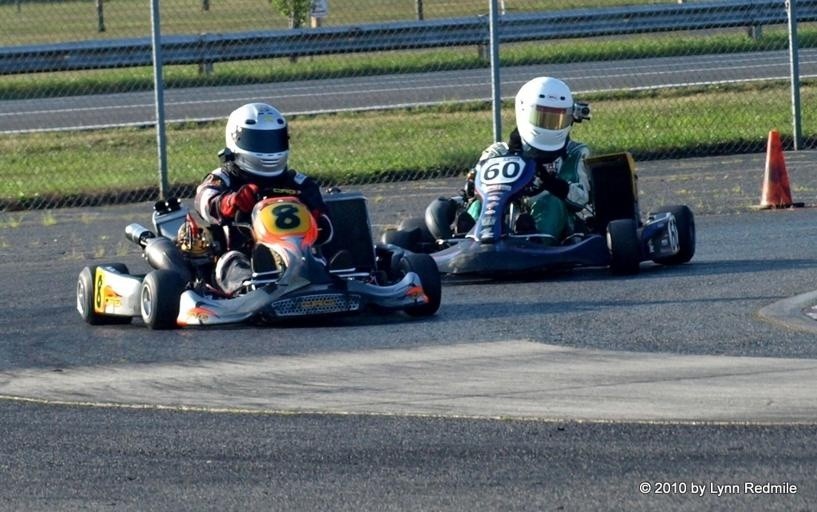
[748, 129, 806, 209]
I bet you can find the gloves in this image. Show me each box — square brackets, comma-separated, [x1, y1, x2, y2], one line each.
[220, 183, 260, 217]
[479, 142, 508, 159]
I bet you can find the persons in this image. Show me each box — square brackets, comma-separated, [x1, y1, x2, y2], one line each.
[470, 140, 588, 237]
[193, 164, 334, 288]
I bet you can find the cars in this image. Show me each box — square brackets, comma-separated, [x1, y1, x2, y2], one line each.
[75, 150, 696, 332]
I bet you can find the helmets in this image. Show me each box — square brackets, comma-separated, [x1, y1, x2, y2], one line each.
[225, 103, 289, 177]
[515, 77, 573, 151]
